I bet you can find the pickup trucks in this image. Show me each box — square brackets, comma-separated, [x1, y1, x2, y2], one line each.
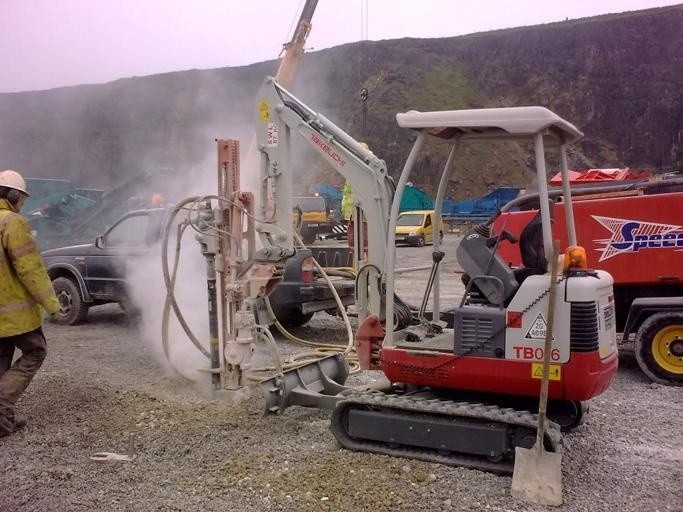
[35, 207, 359, 329]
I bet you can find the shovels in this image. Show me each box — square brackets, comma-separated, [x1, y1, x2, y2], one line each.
[510, 239, 563, 506]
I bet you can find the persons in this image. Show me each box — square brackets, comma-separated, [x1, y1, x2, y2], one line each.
[340, 141, 368, 263]
[0, 170, 62, 437]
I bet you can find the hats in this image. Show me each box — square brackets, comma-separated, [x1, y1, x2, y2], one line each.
[0, 170, 30, 197]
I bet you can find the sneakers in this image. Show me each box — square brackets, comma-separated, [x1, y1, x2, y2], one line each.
[0, 418, 28, 437]
[0, 405, 17, 433]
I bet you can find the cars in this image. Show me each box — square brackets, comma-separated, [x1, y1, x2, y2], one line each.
[390, 210, 444, 245]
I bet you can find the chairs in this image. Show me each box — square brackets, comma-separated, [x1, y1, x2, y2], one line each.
[460, 198, 555, 310]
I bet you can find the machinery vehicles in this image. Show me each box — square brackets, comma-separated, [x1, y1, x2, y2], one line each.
[244, 0, 330, 242]
[456, 163, 681, 387]
[163, 76, 617, 479]
